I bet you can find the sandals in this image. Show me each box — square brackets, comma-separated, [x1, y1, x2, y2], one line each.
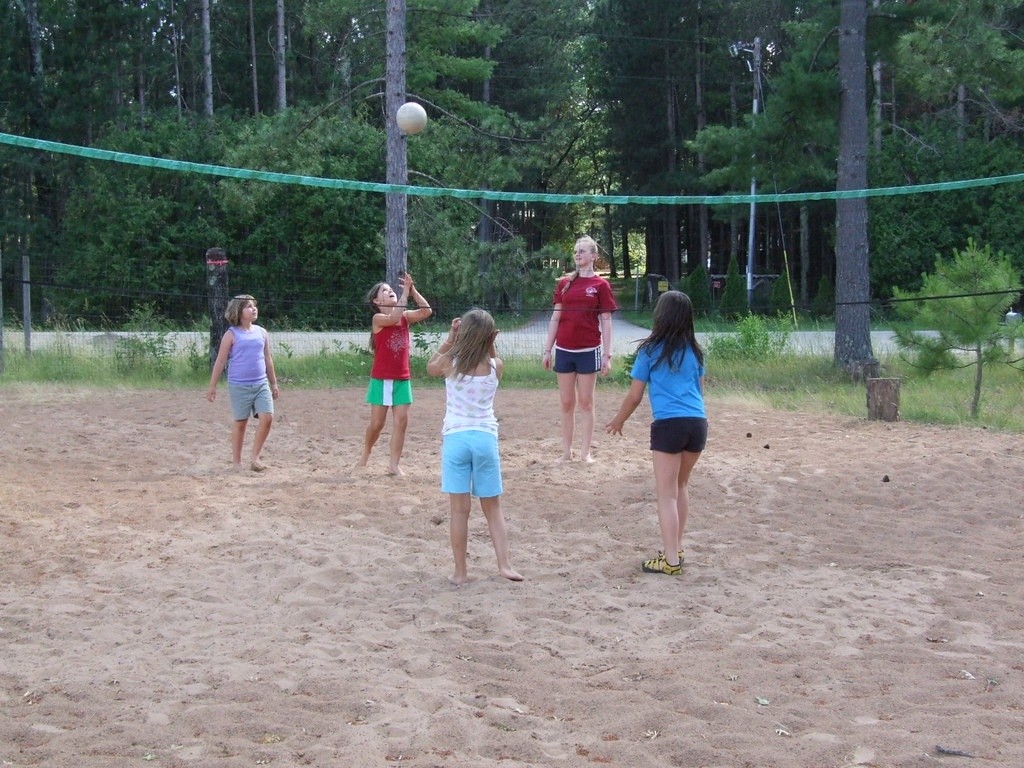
[642, 555, 681, 576]
[658, 549, 685, 563]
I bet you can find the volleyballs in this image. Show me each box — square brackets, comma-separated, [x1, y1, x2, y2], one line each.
[397, 101, 427, 134]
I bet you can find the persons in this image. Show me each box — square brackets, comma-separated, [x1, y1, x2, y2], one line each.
[605, 291, 708, 575]
[428, 308, 524, 585]
[207, 295, 279, 473]
[354, 270, 433, 477]
[542, 235, 618, 470]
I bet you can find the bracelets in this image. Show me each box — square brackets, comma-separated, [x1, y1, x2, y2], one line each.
[444, 341, 452, 346]
[603, 354, 612, 358]
[545, 350, 551, 352]
